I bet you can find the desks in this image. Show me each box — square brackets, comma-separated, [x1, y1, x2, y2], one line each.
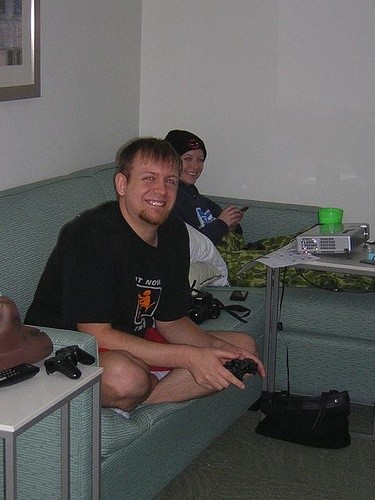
[263, 239, 375, 390]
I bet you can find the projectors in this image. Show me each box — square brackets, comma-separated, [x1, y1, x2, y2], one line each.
[296, 222, 370, 254]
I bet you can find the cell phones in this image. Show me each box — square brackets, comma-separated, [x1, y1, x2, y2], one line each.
[230, 290, 248, 301]
[241, 205, 249, 212]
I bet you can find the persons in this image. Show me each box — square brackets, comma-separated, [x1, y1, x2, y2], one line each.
[162, 130, 375, 292]
[24, 138, 257, 413]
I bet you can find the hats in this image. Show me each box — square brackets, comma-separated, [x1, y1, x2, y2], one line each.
[165, 130, 207, 162]
[0, 295, 54, 372]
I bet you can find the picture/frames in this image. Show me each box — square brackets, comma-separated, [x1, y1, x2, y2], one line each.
[0, 0, 40, 102]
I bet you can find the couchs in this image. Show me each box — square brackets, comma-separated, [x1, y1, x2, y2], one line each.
[0, 156, 375, 500]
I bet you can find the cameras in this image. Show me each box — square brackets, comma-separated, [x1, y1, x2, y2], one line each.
[187, 292, 221, 324]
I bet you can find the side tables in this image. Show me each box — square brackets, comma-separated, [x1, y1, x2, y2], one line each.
[0, 358, 104, 500]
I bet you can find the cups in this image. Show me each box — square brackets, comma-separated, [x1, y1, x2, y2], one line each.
[318, 208, 344, 224]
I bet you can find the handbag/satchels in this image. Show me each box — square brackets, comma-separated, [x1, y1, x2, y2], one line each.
[256, 391, 351, 450]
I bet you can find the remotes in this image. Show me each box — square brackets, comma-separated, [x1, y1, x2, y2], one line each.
[0, 363, 40, 389]
